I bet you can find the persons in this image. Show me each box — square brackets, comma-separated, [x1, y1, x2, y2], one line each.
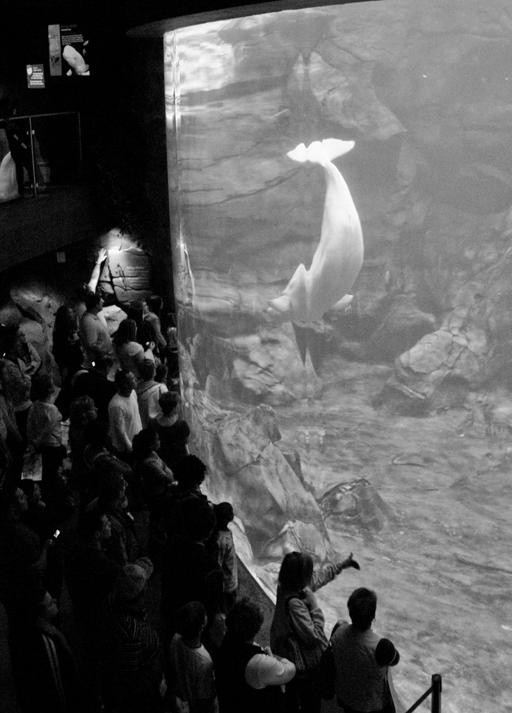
[0, 249, 399, 713]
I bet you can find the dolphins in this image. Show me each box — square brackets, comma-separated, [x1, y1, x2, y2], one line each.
[263, 136, 368, 332]
